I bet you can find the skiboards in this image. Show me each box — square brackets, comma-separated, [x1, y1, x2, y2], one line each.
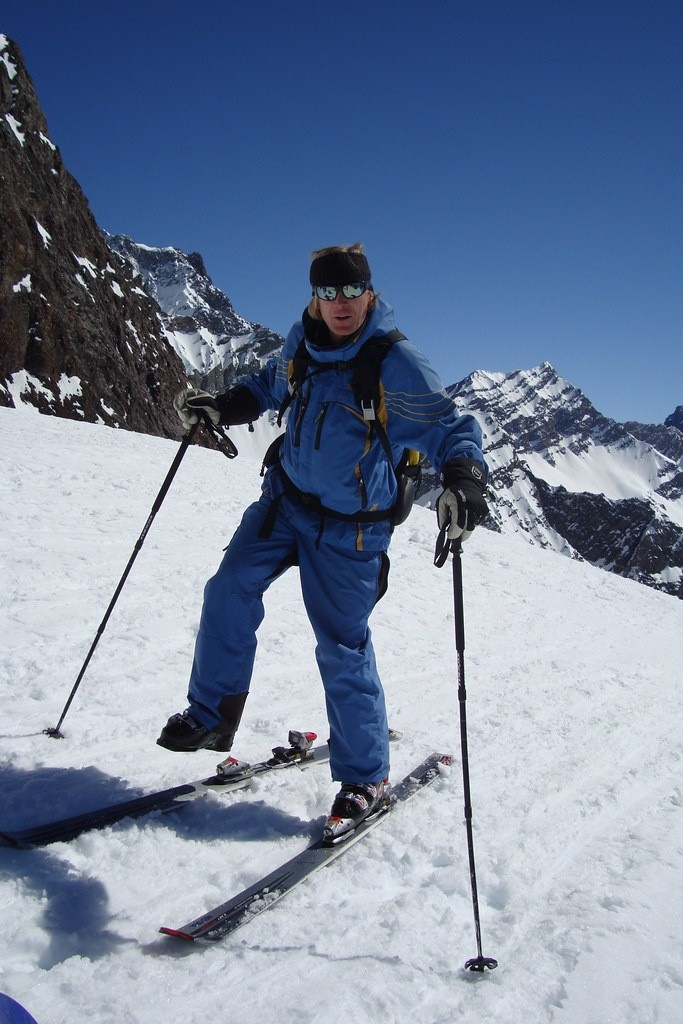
[155, 752, 457, 941]
[0, 724, 403, 852]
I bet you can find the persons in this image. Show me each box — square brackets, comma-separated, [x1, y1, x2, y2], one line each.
[154, 242, 489, 846]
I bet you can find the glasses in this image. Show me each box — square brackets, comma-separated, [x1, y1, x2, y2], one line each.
[315, 281, 366, 301]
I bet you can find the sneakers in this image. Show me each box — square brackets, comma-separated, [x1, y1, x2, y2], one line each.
[156, 709, 235, 752]
[332, 781, 385, 817]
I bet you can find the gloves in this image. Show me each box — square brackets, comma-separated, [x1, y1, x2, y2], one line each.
[436, 457, 489, 543]
[173, 382, 260, 432]
